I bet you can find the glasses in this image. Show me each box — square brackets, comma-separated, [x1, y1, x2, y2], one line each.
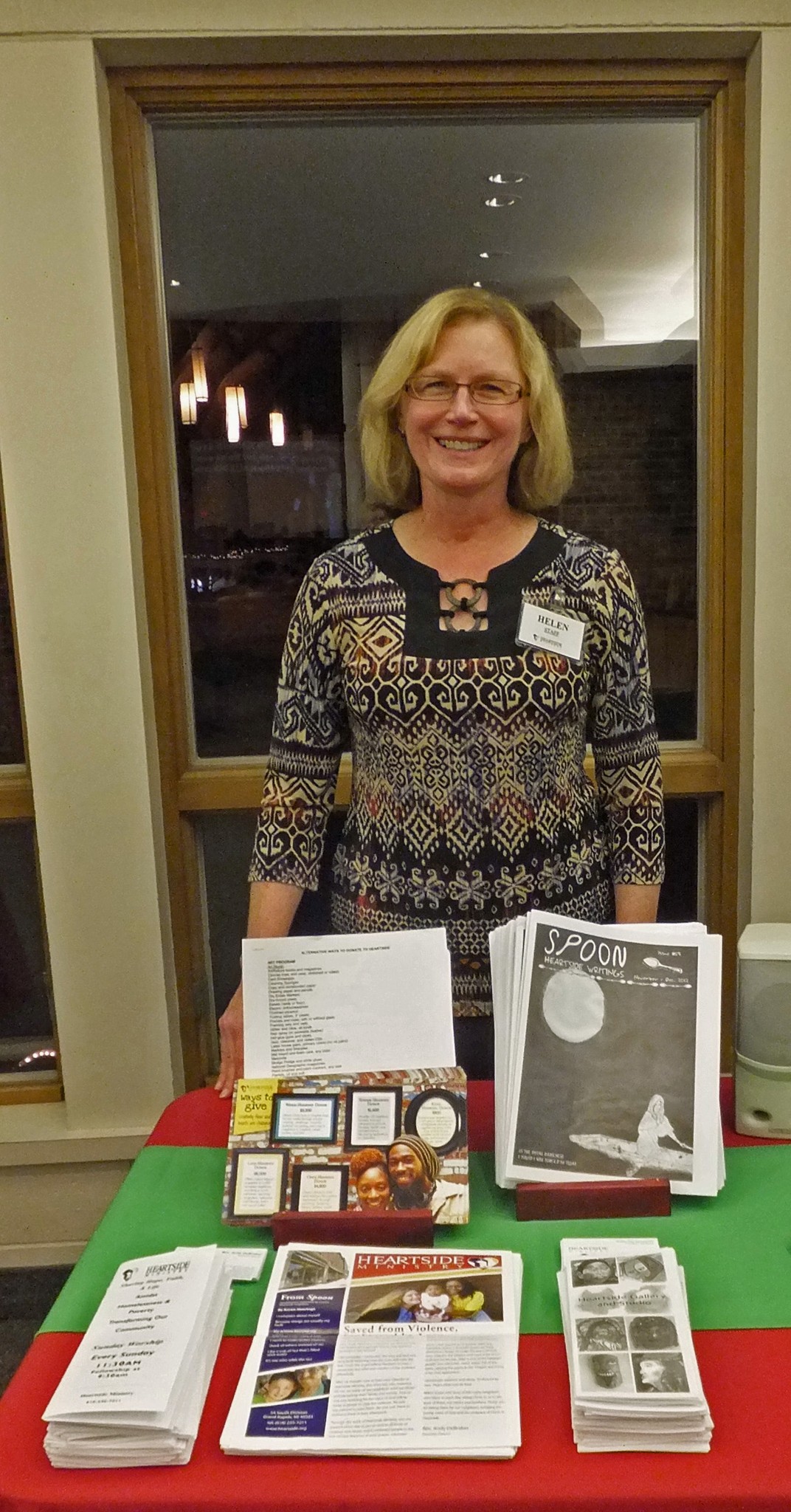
[403, 378, 530, 404]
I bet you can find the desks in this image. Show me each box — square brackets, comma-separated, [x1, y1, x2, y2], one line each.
[0, 1077, 791, 1512]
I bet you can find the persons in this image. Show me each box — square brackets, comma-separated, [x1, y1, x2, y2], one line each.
[634, 1352, 689, 1392]
[395, 1278, 492, 1323]
[252, 1365, 330, 1405]
[350, 1148, 391, 1212]
[216, 289, 664, 1100]
[577, 1259, 618, 1286]
[386, 1134, 468, 1224]
